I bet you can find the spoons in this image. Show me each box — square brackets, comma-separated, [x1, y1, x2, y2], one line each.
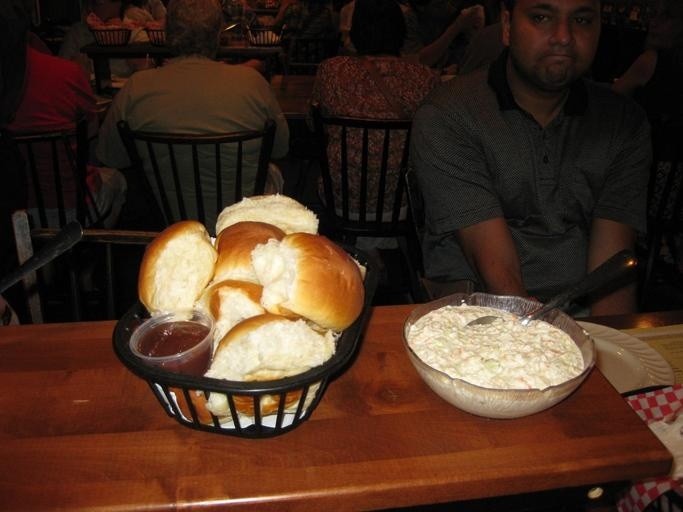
[463, 248, 640, 330]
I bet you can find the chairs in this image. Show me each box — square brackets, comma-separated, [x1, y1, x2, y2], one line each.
[309, 100, 432, 306]
[3, 122, 105, 306]
[38, 3, 344, 74]
[115, 115, 282, 235]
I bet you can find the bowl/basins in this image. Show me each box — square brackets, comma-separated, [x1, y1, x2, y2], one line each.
[400, 290, 597, 419]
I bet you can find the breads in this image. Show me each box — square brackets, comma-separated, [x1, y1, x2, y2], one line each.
[138, 193, 366, 426]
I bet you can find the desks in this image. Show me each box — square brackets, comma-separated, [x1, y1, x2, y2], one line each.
[269, 73, 319, 114]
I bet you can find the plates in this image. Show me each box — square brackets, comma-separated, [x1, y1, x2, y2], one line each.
[576, 319, 676, 395]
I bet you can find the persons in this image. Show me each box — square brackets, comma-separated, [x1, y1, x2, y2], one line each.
[313, 2, 446, 281]
[79, 2, 485, 77]
[593, 2, 682, 288]
[101, 1, 299, 235]
[2, 1, 128, 299]
[409, 0, 648, 321]
